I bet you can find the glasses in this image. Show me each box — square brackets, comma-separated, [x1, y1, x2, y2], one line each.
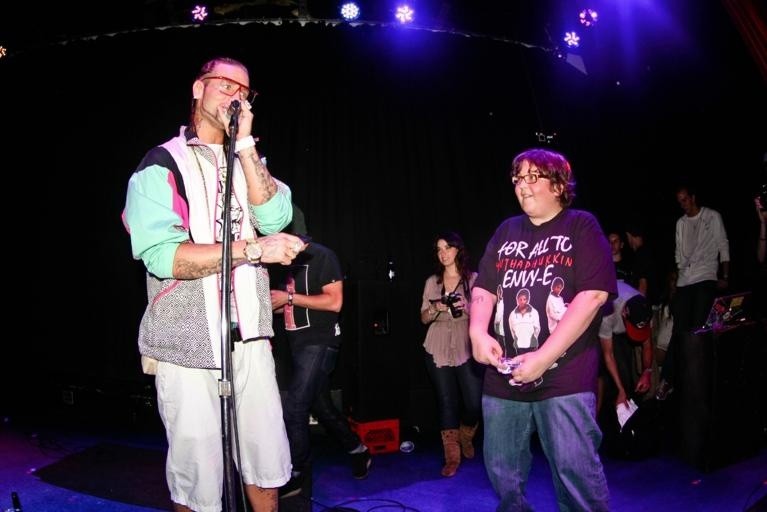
[512, 174, 551, 184]
[201, 76, 258, 105]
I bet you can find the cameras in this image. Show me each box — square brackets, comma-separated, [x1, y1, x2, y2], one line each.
[440, 291, 464, 319]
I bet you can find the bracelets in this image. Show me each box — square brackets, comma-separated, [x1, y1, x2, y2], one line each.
[234, 135, 260, 154]
[428, 306, 437, 316]
[288, 292, 293, 306]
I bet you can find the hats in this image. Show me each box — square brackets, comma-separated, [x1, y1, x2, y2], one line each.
[622, 294, 653, 342]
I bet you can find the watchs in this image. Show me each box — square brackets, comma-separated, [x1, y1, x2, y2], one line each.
[243, 239, 263, 264]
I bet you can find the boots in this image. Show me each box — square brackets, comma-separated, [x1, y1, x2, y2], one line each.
[441, 429, 461, 477]
[460, 423, 479, 459]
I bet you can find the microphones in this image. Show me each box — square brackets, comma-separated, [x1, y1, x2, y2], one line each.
[224, 99, 240, 122]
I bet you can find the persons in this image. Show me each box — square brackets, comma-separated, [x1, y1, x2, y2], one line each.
[120, 56, 310, 511]
[598, 180, 767, 414]
[270, 203, 372, 501]
[494, 284, 508, 357]
[420, 231, 482, 477]
[546, 276, 571, 358]
[468, 149, 619, 511]
[508, 289, 545, 388]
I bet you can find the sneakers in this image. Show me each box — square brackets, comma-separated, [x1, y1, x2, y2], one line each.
[655, 384, 674, 400]
[278, 473, 301, 499]
[349, 445, 371, 480]
